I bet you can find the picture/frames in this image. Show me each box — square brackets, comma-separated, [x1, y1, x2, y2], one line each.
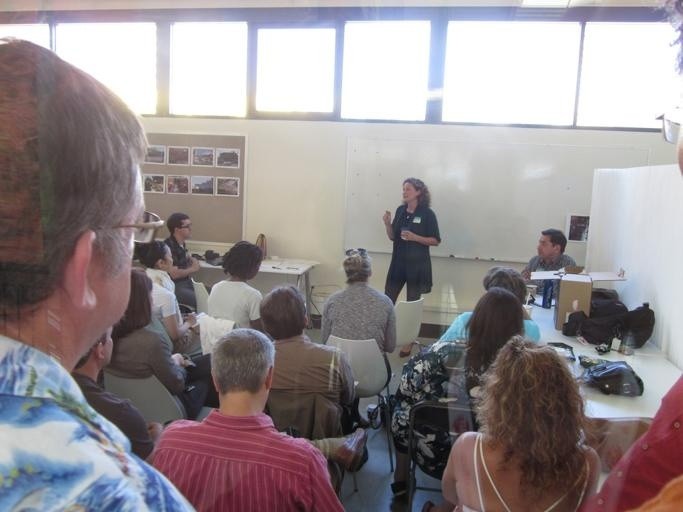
[142, 145, 241, 198]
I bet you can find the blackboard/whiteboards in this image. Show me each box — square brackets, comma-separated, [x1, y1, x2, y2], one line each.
[343, 134, 652, 266]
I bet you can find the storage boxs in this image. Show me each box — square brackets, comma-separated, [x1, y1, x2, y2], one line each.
[530, 270, 628, 331]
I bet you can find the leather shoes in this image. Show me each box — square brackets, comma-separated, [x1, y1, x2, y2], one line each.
[334, 428, 368, 471]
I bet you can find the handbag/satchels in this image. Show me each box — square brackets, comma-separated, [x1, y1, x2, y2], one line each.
[379, 394, 394, 425]
[575, 360, 643, 397]
[563, 288, 655, 348]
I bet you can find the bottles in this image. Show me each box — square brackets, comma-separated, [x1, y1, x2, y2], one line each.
[367, 404, 378, 419]
[542, 279, 554, 308]
[621, 328, 634, 355]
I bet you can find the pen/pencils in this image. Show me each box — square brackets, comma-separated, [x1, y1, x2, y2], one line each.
[286, 267, 299, 270]
[272, 266, 281, 270]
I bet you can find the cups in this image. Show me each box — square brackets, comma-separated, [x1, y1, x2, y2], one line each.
[385, 207, 392, 216]
[526, 285, 537, 297]
[399, 227, 410, 241]
[521, 305, 532, 316]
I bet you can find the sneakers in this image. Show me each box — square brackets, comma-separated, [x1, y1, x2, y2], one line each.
[391, 478, 416, 497]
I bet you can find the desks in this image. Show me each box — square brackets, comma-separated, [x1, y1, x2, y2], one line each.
[198, 256, 321, 329]
[522, 293, 683, 425]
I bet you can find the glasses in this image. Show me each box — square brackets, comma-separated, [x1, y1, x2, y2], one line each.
[91, 210, 165, 244]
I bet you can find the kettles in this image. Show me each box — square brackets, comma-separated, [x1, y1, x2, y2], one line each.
[256, 235, 265, 259]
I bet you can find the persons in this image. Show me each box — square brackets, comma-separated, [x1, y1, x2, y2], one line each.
[73, 243, 263, 460]
[143, 327, 368, 512]
[582, 374, 682, 512]
[260, 285, 358, 439]
[518, 228, 582, 289]
[393, 267, 541, 480]
[1, 36, 200, 509]
[383, 178, 441, 356]
[443, 335, 599, 512]
[159, 213, 205, 306]
[322, 248, 397, 354]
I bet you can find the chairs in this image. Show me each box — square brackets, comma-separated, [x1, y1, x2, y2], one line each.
[290, 422, 359, 494]
[394, 298, 425, 351]
[102, 369, 188, 426]
[431, 340, 467, 376]
[323, 334, 394, 472]
[407, 401, 478, 511]
[190, 277, 209, 314]
